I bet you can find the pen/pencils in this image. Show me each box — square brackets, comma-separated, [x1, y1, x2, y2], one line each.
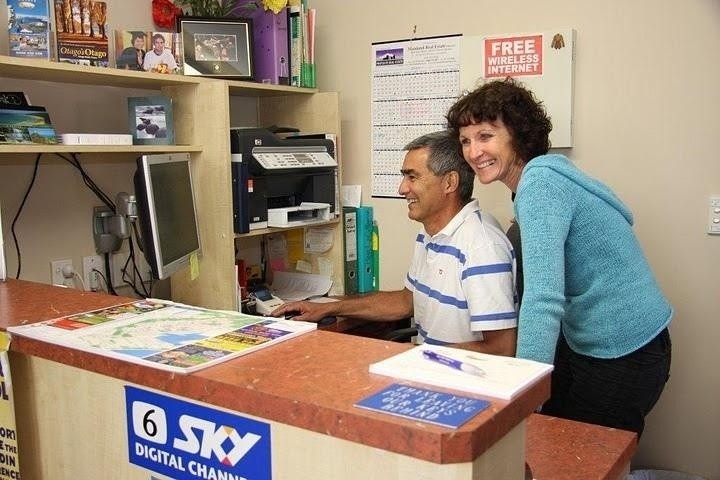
[264, 261, 267, 283]
[420, 349, 492, 378]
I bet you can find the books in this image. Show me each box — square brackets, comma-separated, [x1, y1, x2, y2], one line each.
[370, 341, 556, 401]
[229, 1, 317, 88]
[340, 184, 380, 294]
[6, 297, 319, 377]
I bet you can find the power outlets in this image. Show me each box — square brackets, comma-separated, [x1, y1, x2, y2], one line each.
[50, 258, 73, 287]
[83, 255, 105, 291]
[139, 251, 155, 282]
[113, 253, 131, 288]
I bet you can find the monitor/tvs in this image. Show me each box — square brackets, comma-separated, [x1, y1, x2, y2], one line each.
[133, 153, 202, 281]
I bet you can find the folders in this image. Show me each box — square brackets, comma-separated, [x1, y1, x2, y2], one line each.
[272, 0, 302, 86]
[343, 206, 374, 293]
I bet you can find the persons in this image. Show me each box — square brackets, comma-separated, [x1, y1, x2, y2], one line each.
[143, 33, 179, 74]
[19, 37, 28, 50]
[196, 37, 237, 62]
[116, 31, 148, 72]
[270, 130, 521, 358]
[447, 72, 673, 448]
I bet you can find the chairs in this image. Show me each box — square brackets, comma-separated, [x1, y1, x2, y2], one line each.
[385, 221, 523, 345]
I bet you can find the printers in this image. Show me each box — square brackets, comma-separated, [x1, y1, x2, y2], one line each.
[230, 124, 339, 234]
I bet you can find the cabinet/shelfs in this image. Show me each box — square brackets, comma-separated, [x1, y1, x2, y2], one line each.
[0, 54, 346, 318]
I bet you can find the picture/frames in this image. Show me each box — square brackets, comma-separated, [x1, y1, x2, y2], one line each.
[128, 95, 174, 146]
[177, 15, 255, 79]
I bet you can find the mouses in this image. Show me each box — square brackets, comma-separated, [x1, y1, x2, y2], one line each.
[278, 312, 301, 319]
